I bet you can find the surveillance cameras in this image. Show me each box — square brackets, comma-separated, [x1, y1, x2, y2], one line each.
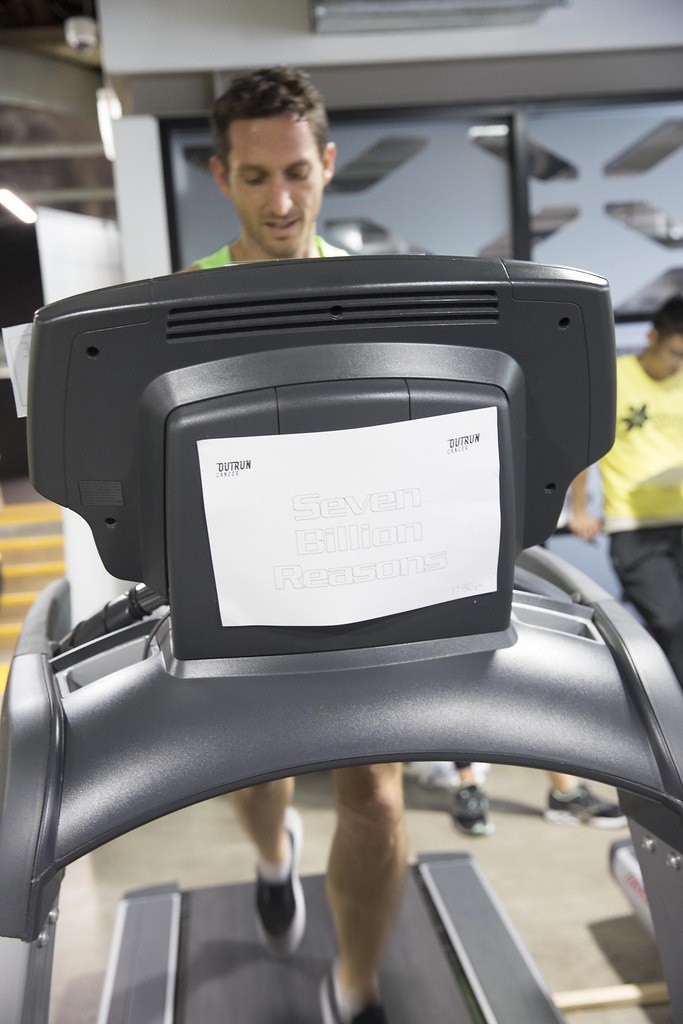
[62, 16, 98, 50]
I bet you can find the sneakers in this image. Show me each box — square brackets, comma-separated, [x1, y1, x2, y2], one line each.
[451, 787, 495, 838]
[317, 970, 388, 1024]
[253, 807, 305, 951]
[544, 785, 629, 827]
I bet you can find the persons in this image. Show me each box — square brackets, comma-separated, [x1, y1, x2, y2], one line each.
[157, 60, 415, 1024]
[561, 290, 683, 687]
